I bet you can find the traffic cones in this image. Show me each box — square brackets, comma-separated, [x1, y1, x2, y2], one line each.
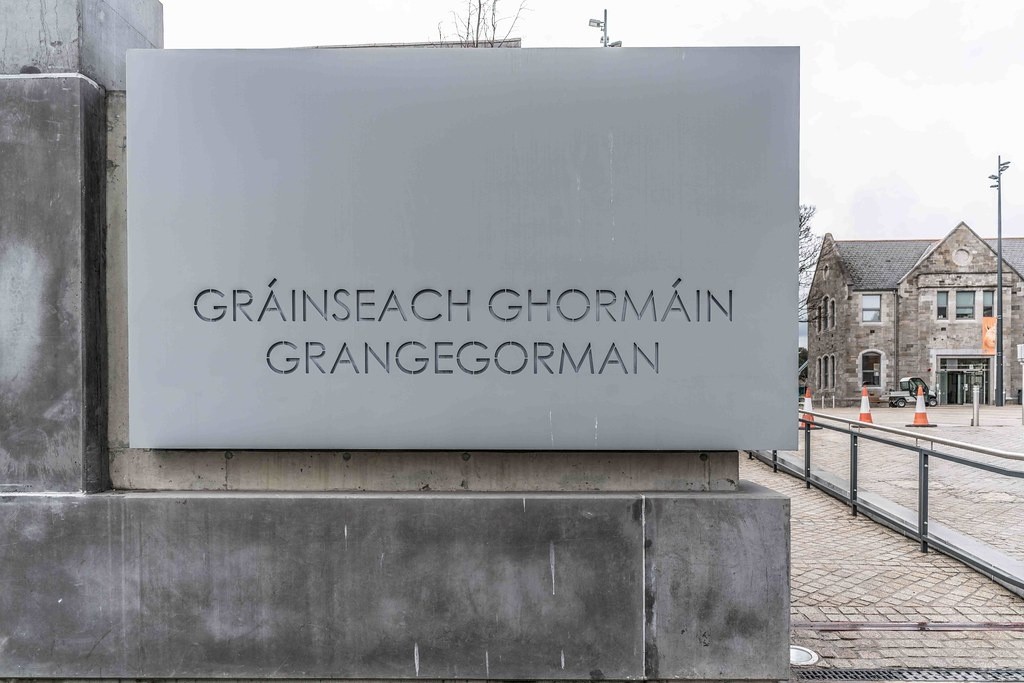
[798, 388, 823, 429]
[905, 384, 937, 427]
[851, 385, 875, 427]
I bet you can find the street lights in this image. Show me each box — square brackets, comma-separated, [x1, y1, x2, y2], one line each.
[588, 9, 622, 47]
[988, 155, 1010, 407]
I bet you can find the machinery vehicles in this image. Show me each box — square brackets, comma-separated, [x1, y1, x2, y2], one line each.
[879, 377, 938, 408]
